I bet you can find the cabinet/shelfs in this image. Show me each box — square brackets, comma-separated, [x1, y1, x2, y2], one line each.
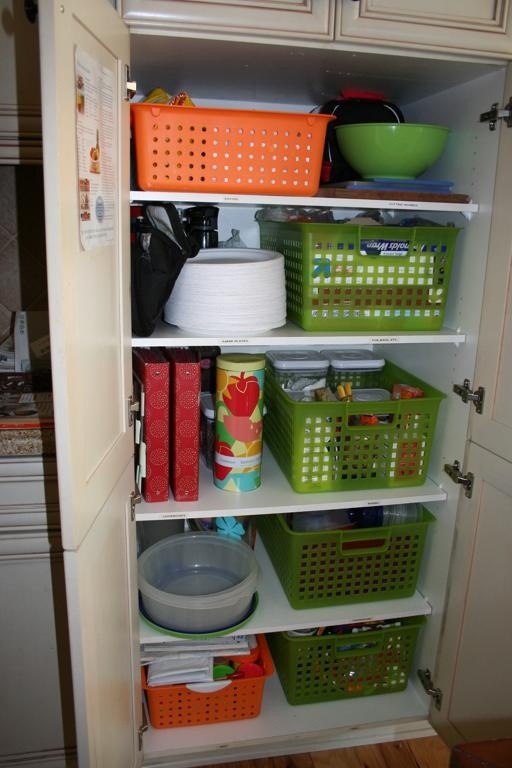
[40, 0, 512, 768]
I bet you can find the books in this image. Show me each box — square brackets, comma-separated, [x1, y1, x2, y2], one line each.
[140, 636, 257, 688]
[0, 311, 54, 420]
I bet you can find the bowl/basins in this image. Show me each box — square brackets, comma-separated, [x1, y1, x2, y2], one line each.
[335, 122, 450, 180]
[137, 530, 261, 632]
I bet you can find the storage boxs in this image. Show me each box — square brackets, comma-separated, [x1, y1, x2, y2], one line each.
[142, 630, 275, 731]
[261, 354, 446, 486]
[129, 96, 335, 197]
[264, 614, 423, 706]
[257, 216, 460, 331]
[258, 503, 435, 613]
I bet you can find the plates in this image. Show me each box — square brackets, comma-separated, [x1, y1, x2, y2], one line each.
[136, 588, 261, 642]
[163, 247, 288, 337]
